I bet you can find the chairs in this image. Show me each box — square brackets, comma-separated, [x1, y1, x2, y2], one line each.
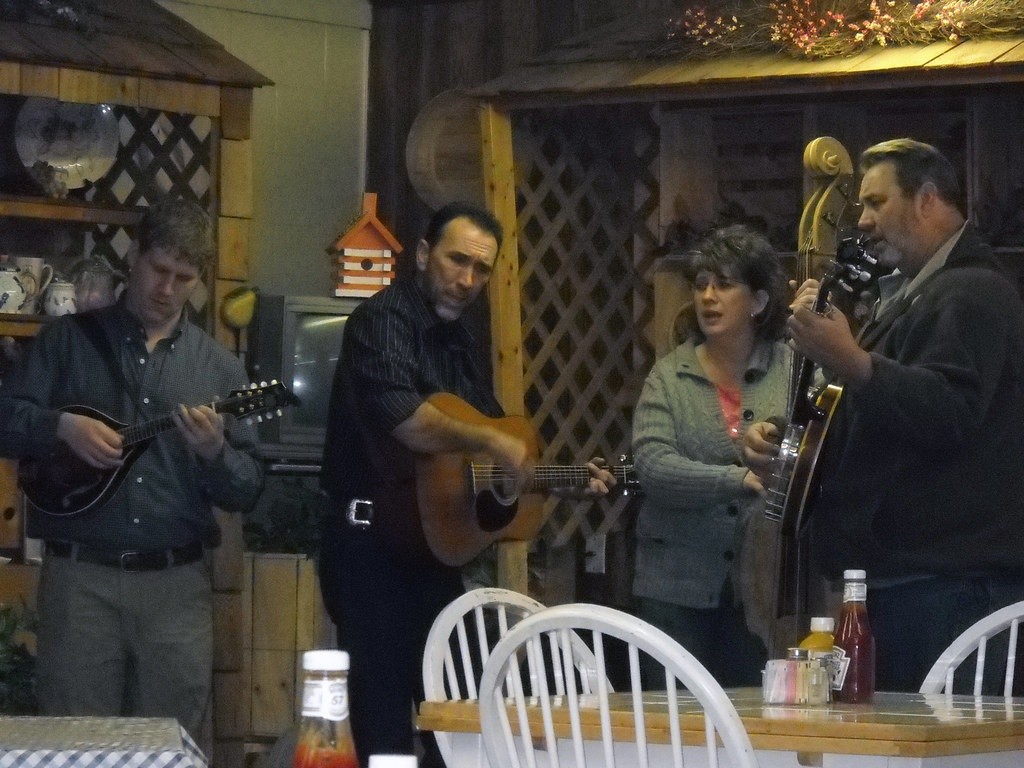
[919, 601, 1024, 698]
[478, 603, 754, 768]
[423, 588, 614, 768]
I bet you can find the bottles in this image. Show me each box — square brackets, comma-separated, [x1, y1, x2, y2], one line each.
[799, 617, 835, 655]
[294, 648, 362, 768]
[805, 657, 829, 706]
[832, 569, 875, 703]
[782, 647, 809, 704]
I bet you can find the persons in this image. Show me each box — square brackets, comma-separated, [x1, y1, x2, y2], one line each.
[742, 140, 1024, 692]
[317, 201, 616, 768]
[627, 224, 830, 692]
[0, 201, 269, 768]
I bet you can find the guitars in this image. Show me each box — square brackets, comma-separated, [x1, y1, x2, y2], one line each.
[764, 232, 879, 541]
[16, 379, 291, 519]
[366, 389, 641, 568]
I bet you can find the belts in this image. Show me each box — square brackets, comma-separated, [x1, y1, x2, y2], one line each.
[42, 539, 205, 573]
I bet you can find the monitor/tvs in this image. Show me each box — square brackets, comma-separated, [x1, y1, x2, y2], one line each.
[247, 293, 367, 465]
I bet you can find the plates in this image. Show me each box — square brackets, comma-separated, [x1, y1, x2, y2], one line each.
[14, 93, 119, 190]
[405, 85, 533, 217]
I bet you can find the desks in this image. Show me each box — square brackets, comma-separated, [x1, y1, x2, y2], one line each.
[418, 687, 1024, 768]
[0, 716, 207, 768]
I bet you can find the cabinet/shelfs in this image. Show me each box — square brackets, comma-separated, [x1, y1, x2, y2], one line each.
[2, 62, 224, 566]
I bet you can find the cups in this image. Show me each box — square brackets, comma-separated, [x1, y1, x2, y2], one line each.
[11, 256, 53, 314]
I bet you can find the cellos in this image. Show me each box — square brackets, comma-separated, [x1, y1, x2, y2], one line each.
[732, 135, 858, 674]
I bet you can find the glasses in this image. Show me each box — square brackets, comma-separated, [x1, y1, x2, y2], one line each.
[690, 275, 753, 298]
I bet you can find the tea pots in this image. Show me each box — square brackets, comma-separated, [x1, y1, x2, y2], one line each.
[43, 277, 79, 315]
[0, 253, 40, 314]
